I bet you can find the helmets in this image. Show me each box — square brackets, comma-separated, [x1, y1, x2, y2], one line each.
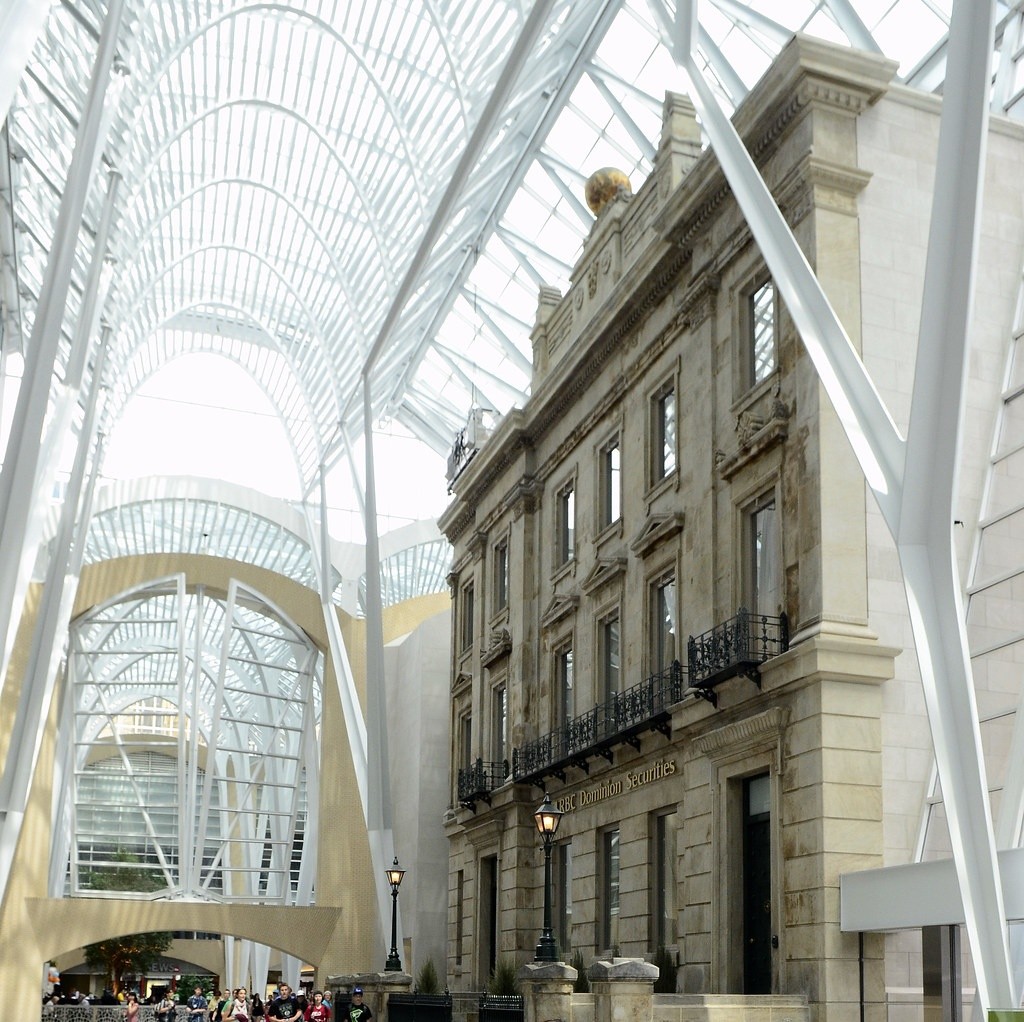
[296, 989, 305, 997]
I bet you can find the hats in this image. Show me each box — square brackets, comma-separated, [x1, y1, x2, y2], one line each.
[354, 987, 363, 996]
[323, 991, 332, 1000]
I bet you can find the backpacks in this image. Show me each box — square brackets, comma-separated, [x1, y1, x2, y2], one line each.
[154, 999, 167, 1018]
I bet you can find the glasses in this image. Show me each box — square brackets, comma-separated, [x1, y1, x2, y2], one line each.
[353, 993, 363, 996]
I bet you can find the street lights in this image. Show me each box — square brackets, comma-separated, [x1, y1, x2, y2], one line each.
[383, 855, 407, 970]
[532, 790, 565, 960]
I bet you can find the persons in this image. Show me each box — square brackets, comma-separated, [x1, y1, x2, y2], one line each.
[77, 994, 90, 1005]
[117, 989, 128, 1002]
[70, 986, 80, 1005]
[265, 982, 373, 1022]
[208, 988, 265, 1022]
[122, 992, 141, 1022]
[42, 996, 59, 1017]
[158, 988, 176, 1022]
[186, 986, 208, 1022]
